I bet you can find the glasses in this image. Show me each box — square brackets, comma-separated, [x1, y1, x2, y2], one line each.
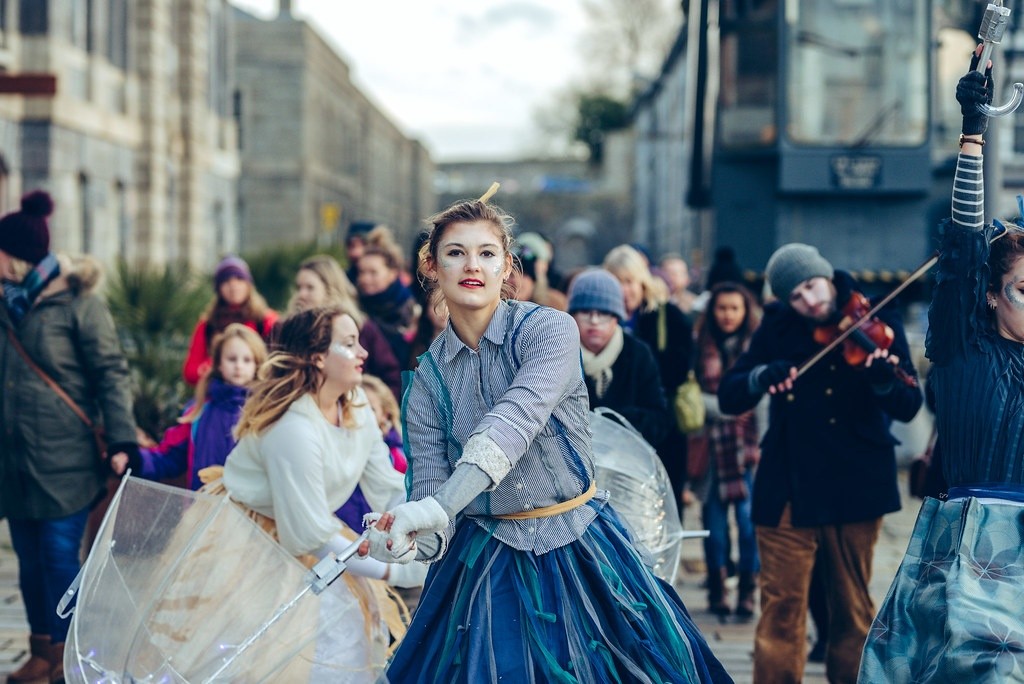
[577, 310, 613, 325]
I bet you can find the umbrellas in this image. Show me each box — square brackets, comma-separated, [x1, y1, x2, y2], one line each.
[588, 407, 710, 585]
[976, 0, 1024, 117]
[55, 468, 416, 684]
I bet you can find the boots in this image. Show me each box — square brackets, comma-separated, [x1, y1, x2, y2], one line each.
[5, 635, 65, 684]
[705, 565, 730, 615]
[736, 571, 757, 622]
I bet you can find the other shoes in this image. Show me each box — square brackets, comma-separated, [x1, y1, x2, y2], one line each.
[808, 640, 826, 663]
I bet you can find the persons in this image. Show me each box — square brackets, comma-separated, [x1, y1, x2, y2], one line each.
[0, 188, 143, 684]
[113, 200, 924, 684]
[857, 43, 1024, 684]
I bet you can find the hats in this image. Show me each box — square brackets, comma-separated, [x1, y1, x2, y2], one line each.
[509, 243, 540, 285]
[0, 190, 54, 265]
[213, 253, 251, 290]
[766, 245, 834, 305]
[566, 266, 628, 325]
[344, 219, 376, 243]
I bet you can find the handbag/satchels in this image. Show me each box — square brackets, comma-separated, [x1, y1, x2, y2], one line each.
[676, 380, 705, 437]
[93, 420, 159, 464]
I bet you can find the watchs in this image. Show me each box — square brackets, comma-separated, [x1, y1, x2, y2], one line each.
[959, 133, 986, 147]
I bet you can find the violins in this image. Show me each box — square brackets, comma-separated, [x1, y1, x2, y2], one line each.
[813, 291, 919, 389]
